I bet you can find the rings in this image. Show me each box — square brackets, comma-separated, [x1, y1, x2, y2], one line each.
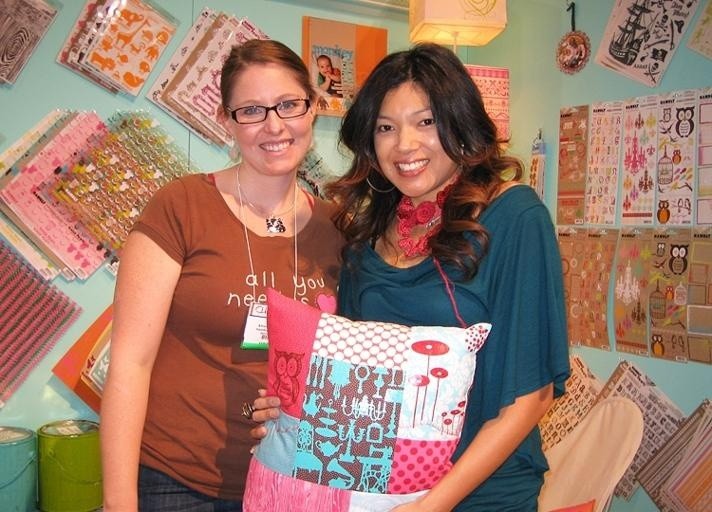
[242, 402, 259, 421]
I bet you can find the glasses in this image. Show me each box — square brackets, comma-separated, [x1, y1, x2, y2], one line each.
[225, 95, 310, 123]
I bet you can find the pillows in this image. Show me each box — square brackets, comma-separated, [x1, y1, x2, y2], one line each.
[242, 286, 492, 512]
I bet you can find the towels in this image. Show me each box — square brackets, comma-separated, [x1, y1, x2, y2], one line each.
[529, 155, 546, 203]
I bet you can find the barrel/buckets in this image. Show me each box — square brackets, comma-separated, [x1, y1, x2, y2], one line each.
[0, 424, 37, 512]
[37, 418, 103, 511]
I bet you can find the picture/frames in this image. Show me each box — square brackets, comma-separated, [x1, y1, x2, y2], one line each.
[555, 30, 592, 75]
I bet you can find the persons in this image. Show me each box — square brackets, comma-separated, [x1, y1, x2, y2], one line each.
[100, 41, 363, 512]
[250, 42, 572, 512]
[316, 54, 342, 100]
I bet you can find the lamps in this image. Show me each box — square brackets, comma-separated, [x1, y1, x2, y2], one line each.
[408, 0, 512, 145]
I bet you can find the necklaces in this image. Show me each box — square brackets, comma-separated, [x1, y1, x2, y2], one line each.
[396, 175, 460, 258]
[242, 184, 299, 236]
